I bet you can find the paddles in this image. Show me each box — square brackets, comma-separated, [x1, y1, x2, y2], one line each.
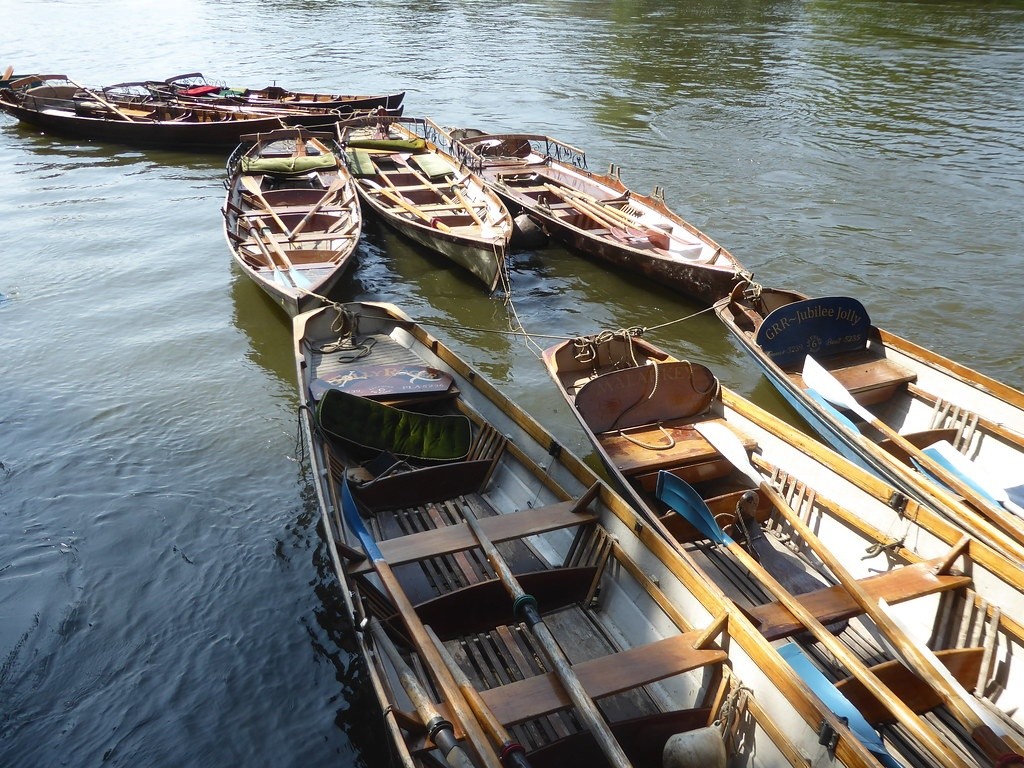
[544, 182, 635, 239]
[1, 66, 11, 79]
[67, 78, 134, 122]
[459, 503, 633, 767]
[551, 184, 652, 238]
[340, 465, 503, 766]
[242, 216, 292, 290]
[257, 216, 312, 290]
[804, 388, 1024, 561]
[559, 186, 670, 251]
[694, 423, 1022, 766]
[445, 175, 503, 238]
[288, 179, 345, 240]
[572, 191, 702, 245]
[656, 469, 972, 768]
[801, 353, 1024, 544]
[390, 154, 457, 213]
[240, 176, 290, 236]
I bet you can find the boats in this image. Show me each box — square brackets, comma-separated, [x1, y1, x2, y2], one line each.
[220, 124, 362, 320]
[0, 64, 406, 149]
[714, 281, 1024, 571]
[437, 125, 754, 302]
[293, 301, 888, 768]
[335, 116, 513, 292]
[541, 326, 1024, 768]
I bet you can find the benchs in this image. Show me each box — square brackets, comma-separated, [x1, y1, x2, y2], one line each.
[593, 412, 758, 476]
[237, 228, 358, 248]
[778, 347, 917, 395]
[346, 498, 600, 574]
[743, 555, 973, 642]
[510, 185, 558, 193]
[366, 182, 465, 193]
[398, 628, 728, 753]
[490, 166, 549, 176]
[385, 202, 487, 213]
[238, 205, 352, 216]
[368, 385, 461, 408]
[539, 197, 630, 217]
[244, 164, 338, 176]
[584, 224, 672, 235]
